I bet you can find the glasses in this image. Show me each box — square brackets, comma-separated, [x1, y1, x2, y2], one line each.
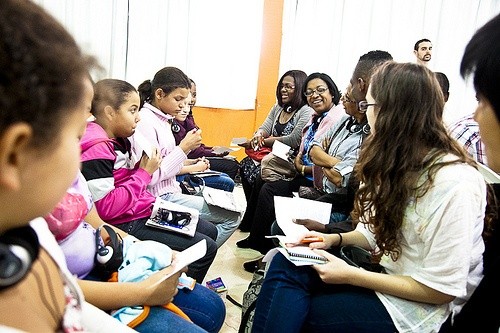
[345, 92, 356, 103]
[280, 84, 295, 91]
[304, 87, 329, 97]
[359, 101, 377, 112]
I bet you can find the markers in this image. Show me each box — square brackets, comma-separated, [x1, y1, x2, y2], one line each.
[254, 136, 264, 151]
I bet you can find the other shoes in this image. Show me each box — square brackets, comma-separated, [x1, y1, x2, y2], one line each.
[237, 238, 252, 248]
[244, 257, 260, 273]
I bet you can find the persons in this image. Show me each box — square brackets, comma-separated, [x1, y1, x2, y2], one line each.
[0, 0, 500, 333]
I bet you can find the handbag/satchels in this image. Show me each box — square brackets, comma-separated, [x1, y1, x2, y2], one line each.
[245, 144, 271, 161]
[260, 152, 297, 181]
[176, 170, 235, 196]
[327, 243, 383, 274]
[242, 266, 267, 333]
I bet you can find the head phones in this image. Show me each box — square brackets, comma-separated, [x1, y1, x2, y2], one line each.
[181, 174, 205, 196]
[96, 224, 123, 272]
[312, 111, 329, 124]
[0, 225, 39, 290]
[340, 244, 383, 273]
[283, 104, 294, 113]
[160, 208, 191, 226]
[345, 116, 371, 135]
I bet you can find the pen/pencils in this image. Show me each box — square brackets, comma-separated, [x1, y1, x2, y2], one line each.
[302, 237, 323, 242]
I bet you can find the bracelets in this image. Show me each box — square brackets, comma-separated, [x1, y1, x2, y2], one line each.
[334, 233, 342, 247]
[301, 165, 305, 176]
